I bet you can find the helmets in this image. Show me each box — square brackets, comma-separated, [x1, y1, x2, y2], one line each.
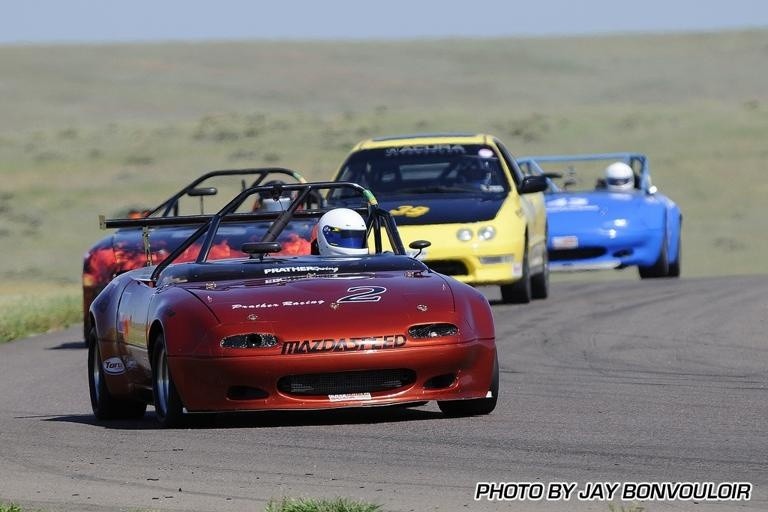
[606, 163, 634, 192]
[258, 181, 292, 212]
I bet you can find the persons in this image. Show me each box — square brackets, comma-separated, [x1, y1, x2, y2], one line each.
[455, 161, 494, 189]
[603, 162, 634, 192]
[254, 181, 293, 210]
